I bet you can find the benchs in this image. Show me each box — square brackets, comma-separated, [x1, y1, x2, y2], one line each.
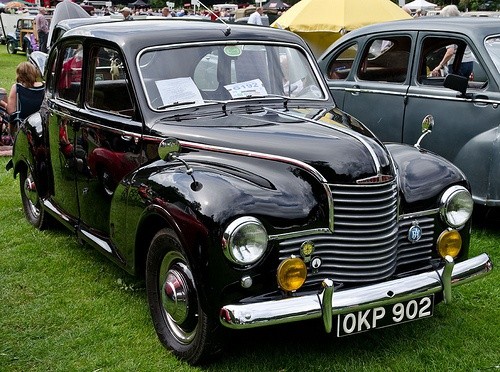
[337, 67, 407, 82]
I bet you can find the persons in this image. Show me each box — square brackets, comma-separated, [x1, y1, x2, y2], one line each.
[224, 10, 232, 16]
[200, 9, 221, 21]
[90, 10, 98, 16]
[190, 11, 195, 15]
[162, 7, 172, 18]
[104, 9, 111, 16]
[247, 7, 263, 26]
[276, 11, 282, 19]
[146, 9, 154, 16]
[433, 4, 474, 83]
[0, 62, 45, 133]
[35, 8, 48, 53]
[120, 7, 134, 21]
[135, 9, 140, 15]
[179, 9, 189, 17]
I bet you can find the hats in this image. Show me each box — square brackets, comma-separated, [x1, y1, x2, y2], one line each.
[119, 7, 131, 13]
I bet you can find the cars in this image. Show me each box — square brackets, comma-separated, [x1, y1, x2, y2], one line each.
[292, 10, 500, 211]
[5, 15, 494, 367]
[7, 17, 51, 58]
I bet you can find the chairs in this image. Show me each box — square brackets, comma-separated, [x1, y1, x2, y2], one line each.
[59, 138, 91, 183]
[0, 84, 45, 146]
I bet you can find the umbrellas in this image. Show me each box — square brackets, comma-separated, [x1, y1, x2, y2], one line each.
[262, 0, 290, 10]
[405, 0, 437, 11]
[270, 0, 415, 58]
